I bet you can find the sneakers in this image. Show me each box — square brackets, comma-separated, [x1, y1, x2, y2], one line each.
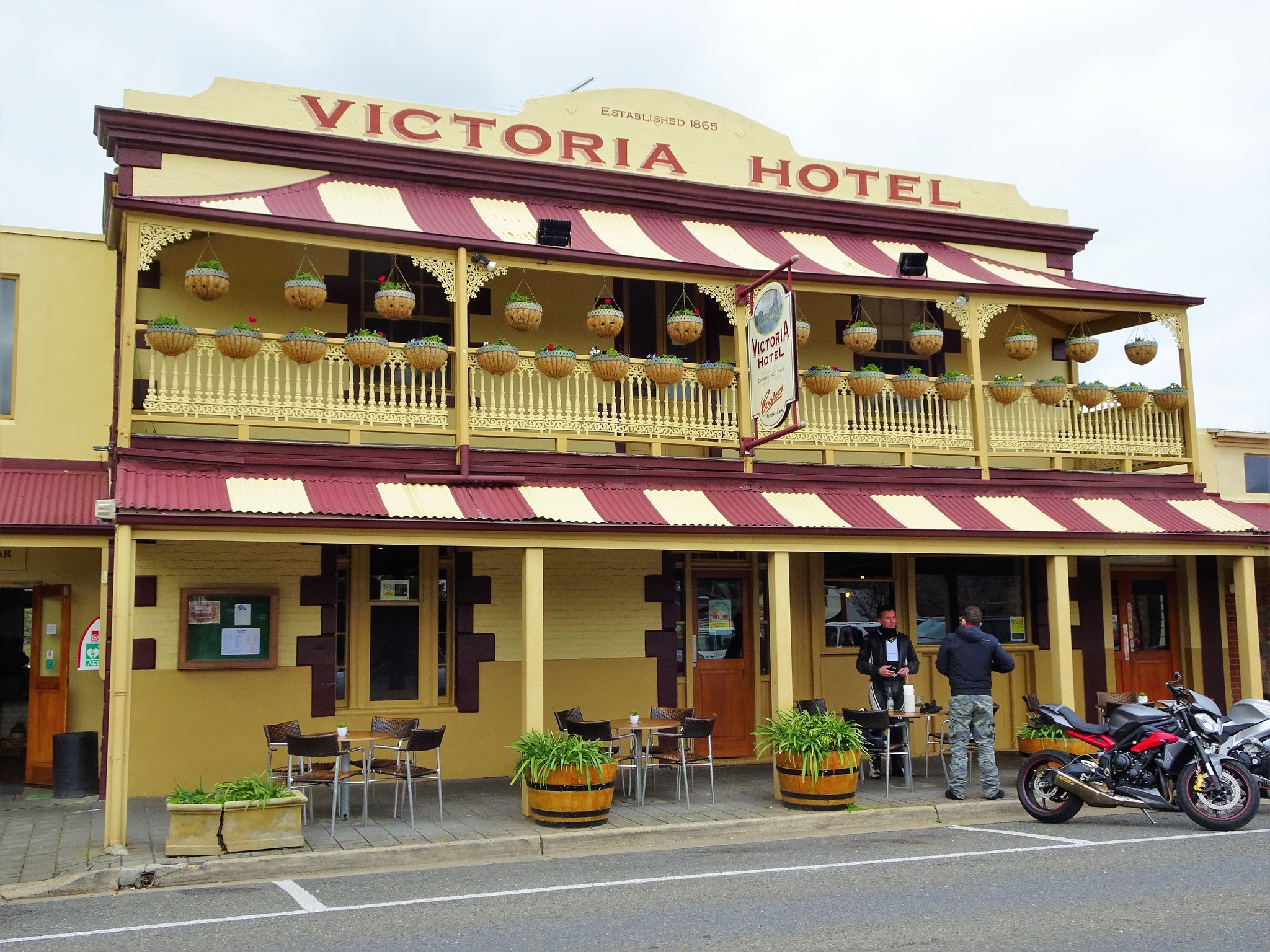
[890, 767, 916, 778]
[868, 759, 881, 779]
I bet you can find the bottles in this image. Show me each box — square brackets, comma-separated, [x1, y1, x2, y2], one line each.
[886, 687, 894, 715]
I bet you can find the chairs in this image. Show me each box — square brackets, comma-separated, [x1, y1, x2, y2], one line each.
[1022, 691, 1155, 723]
[796, 698, 1000, 798]
[554, 706, 717, 810]
[263, 717, 446, 836]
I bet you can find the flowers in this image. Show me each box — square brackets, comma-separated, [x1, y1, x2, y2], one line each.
[145, 232, 1188, 412]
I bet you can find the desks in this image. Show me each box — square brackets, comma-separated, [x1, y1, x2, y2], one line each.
[308, 731, 394, 824]
[889, 711, 946, 787]
[610, 718, 681, 804]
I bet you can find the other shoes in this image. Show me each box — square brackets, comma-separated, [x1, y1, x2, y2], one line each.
[945, 789, 964, 800]
[985, 789, 1004, 800]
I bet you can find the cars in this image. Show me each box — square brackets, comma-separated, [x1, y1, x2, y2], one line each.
[917, 615, 1013, 646]
[676, 633, 731, 669]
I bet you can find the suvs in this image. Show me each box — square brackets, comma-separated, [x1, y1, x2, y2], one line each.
[825, 620, 883, 647]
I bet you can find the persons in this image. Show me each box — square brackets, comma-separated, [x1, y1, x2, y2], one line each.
[935, 604, 1015, 800]
[856, 606, 919, 780]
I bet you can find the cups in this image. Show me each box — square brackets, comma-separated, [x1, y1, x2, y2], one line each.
[903, 694, 915, 713]
[915, 696, 924, 707]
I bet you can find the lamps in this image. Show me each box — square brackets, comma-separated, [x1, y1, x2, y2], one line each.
[890, 252, 929, 278]
[536, 218, 572, 248]
[956, 294, 970, 308]
[471, 253, 497, 272]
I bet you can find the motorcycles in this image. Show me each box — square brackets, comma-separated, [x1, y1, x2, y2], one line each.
[1015, 671, 1260, 831]
[1221, 697, 1270, 802]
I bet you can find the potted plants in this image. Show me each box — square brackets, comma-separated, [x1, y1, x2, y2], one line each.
[1012, 712, 1101, 791]
[503, 727, 618, 829]
[629, 710, 639, 724]
[749, 704, 875, 811]
[915, 703, 922, 712]
[336, 722, 348, 737]
[1137, 692, 1148, 704]
[161, 769, 308, 855]
